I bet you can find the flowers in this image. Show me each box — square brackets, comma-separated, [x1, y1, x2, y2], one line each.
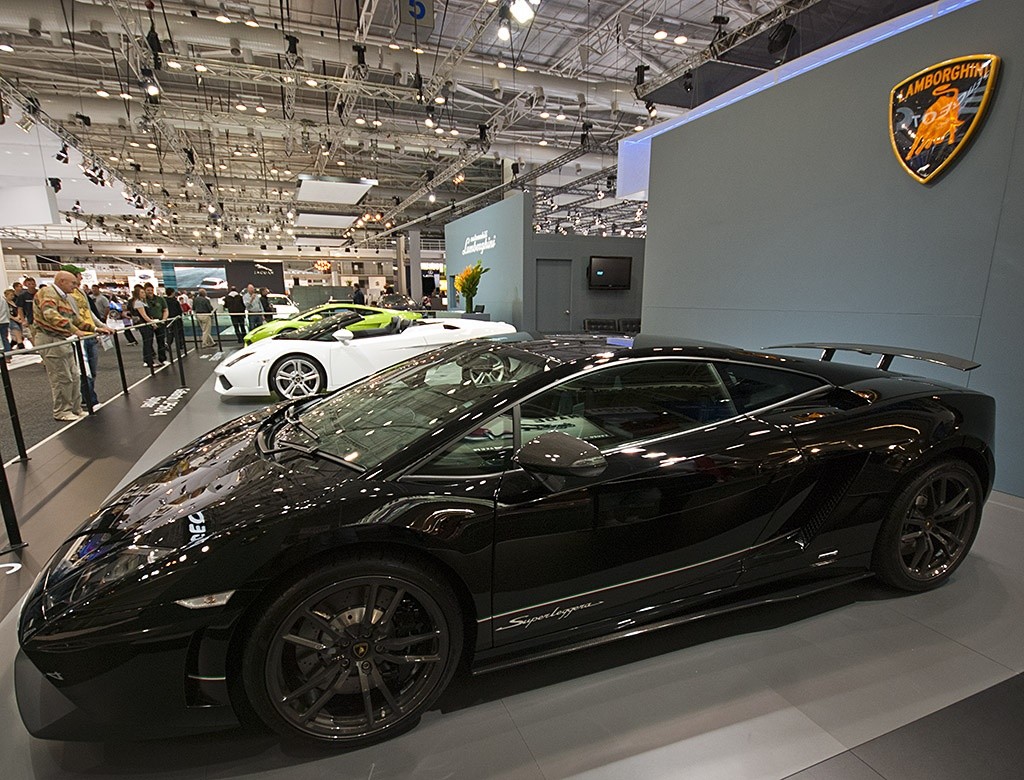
[453, 259, 491, 300]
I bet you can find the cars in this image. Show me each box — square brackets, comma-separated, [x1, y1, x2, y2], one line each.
[377, 293, 420, 311]
[266, 293, 299, 319]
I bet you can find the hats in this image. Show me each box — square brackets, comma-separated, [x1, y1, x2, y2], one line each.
[62, 265, 85, 274]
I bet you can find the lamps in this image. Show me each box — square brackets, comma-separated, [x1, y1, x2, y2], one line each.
[0, 0, 796, 254]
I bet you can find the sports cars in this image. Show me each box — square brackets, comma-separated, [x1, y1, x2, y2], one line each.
[202, 279, 225, 291]
[15, 329, 999, 754]
[242, 299, 423, 349]
[213, 309, 517, 400]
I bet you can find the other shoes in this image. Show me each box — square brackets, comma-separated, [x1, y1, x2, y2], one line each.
[59, 413, 79, 420]
[162, 361, 168, 364]
[77, 411, 88, 416]
[127, 342, 137, 346]
[143, 363, 159, 367]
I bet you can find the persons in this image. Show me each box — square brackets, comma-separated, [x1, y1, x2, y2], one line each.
[60, 265, 116, 410]
[353, 281, 394, 305]
[0, 277, 272, 368]
[33, 270, 110, 421]
